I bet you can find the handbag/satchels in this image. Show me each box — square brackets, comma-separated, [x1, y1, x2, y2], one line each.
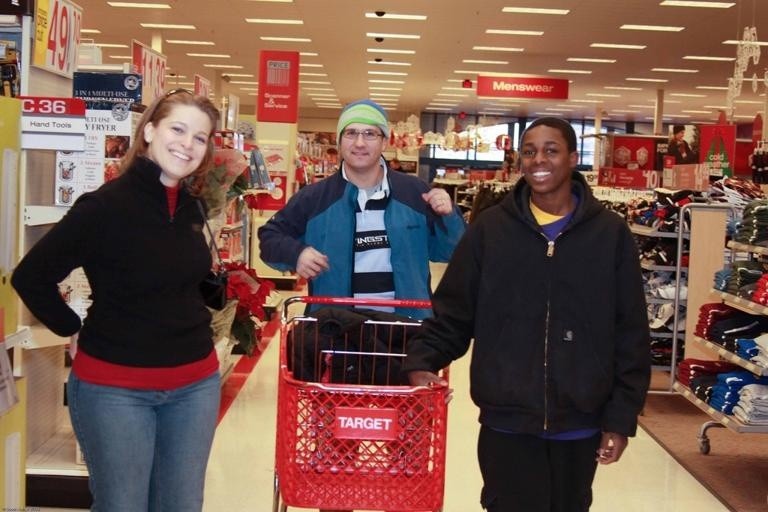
[195, 198, 227, 311]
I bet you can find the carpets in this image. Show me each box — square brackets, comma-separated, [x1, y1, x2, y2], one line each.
[633, 369, 767, 511]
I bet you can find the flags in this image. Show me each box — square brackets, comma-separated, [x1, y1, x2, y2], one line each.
[697, 123, 735, 178]
[257, 50, 299, 123]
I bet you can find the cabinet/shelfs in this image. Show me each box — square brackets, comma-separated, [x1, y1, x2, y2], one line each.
[432, 178, 517, 227]
[3, 103, 418, 510]
[672, 201, 766, 455]
[602, 189, 768, 390]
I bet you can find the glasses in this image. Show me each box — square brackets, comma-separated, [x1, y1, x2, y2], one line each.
[166, 88, 192, 97]
[342, 129, 385, 140]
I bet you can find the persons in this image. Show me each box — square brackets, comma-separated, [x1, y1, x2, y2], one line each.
[666, 124, 696, 163]
[401, 113, 652, 512]
[256, 99, 469, 328]
[9, 90, 225, 512]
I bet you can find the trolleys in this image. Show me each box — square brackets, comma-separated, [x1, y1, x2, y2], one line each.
[272, 292, 451, 511]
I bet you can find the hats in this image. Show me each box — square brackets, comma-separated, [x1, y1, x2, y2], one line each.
[336, 99, 390, 150]
